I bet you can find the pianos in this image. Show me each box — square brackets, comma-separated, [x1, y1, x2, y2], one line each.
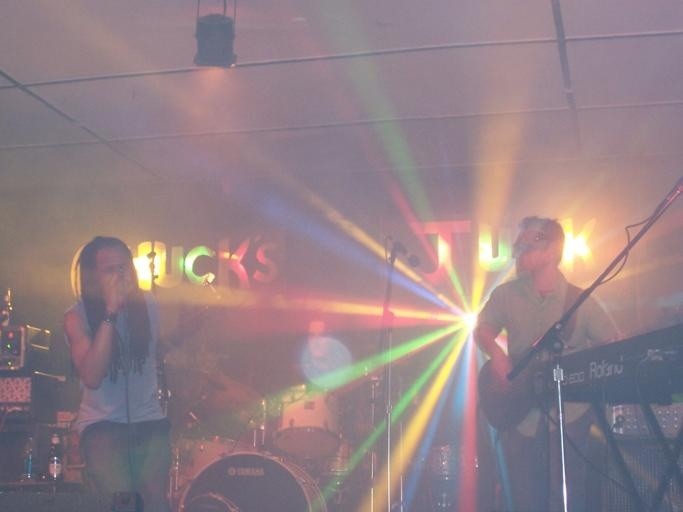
[530, 326, 681, 402]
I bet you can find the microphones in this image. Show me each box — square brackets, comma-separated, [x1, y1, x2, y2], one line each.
[576, 411, 603, 440]
[393, 237, 420, 267]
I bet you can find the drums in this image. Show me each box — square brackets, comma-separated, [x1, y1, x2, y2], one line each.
[177, 452, 326, 511]
[186, 435, 246, 481]
[270, 384, 339, 459]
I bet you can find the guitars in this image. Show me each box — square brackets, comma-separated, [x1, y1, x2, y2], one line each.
[479, 353, 572, 432]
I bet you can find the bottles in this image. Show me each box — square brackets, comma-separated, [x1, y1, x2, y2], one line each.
[1, 285, 16, 328]
[49, 432, 66, 482]
[17, 430, 37, 483]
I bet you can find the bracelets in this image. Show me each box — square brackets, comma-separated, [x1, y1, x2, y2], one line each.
[100, 312, 117, 327]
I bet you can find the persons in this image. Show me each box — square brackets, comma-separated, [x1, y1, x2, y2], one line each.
[62, 235, 207, 509]
[472, 216, 621, 509]
